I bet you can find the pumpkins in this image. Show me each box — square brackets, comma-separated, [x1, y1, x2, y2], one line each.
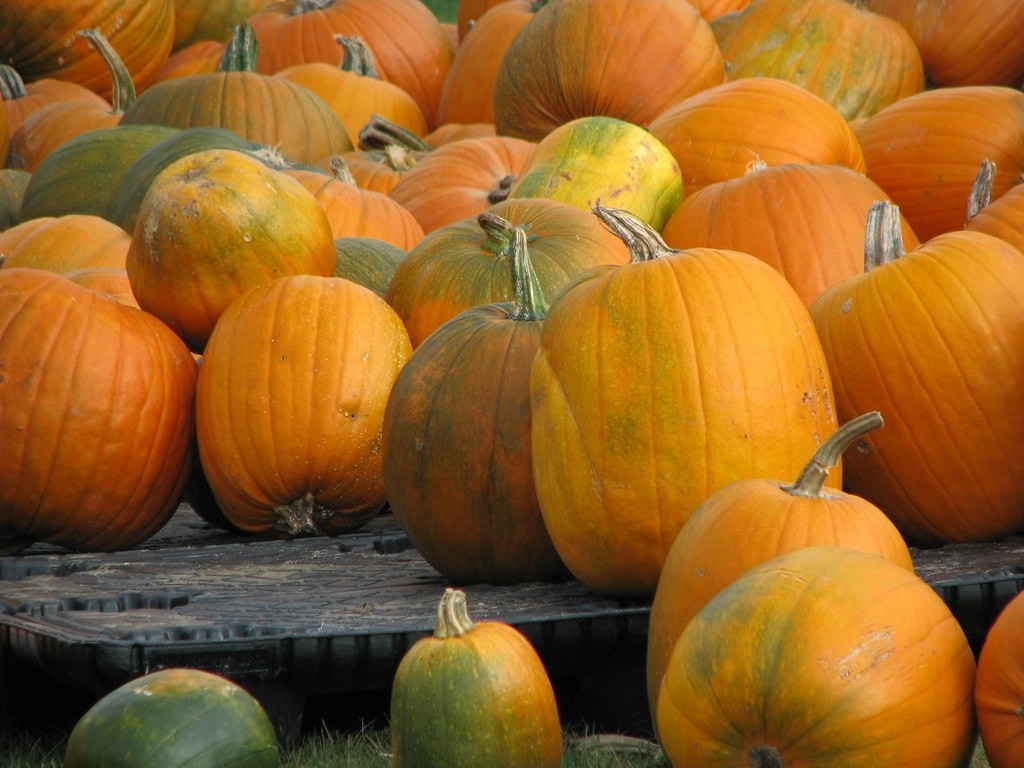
[0, 0, 1024, 768]
